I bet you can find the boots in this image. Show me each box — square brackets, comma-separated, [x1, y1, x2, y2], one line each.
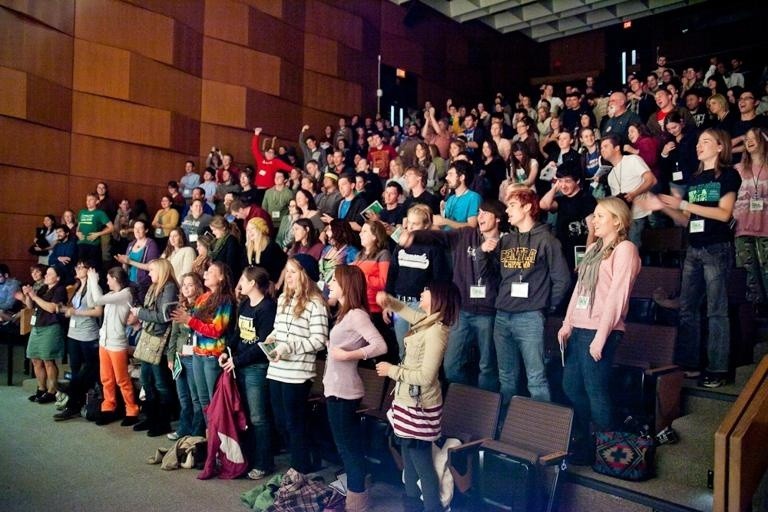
[345, 487, 370, 512]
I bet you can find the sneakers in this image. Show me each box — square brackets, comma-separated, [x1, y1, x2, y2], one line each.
[701, 372, 727, 388]
[38, 390, 58, 404]
[248, 466, 272, 479]
[28, 389, 47, 402]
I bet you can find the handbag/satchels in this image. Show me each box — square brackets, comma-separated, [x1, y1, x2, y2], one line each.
[132, 330, 166, 364]
[629, 296, 659, 327]
[592, 417, 658, 483]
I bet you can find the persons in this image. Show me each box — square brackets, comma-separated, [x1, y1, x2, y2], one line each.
[514, 91, 526, 109]
[421, 107, 452, 160]
[262, 170, 295, 239]
[445, 152, 485, 204]
[635, 130, 741, 389]
[512, 119, 538, 159]
[414, 143, 440, 195]
[275, 198, 302, 252]
[541, 99, 551, 111]
[318, 222, 360, 302]
[172, 264, 236, 470]
[333, 150, 355, 177]
[324, 264, 387, 512]
[33, 215, 58, 268]
[491, 111, 514, 140]
[471, 107, 480, 120]
[352, 126, 368, 157]
[427, 144, 449, 195]
[676, 66, 706, 105]
[440, 140, 470, 197]
[368, 132, 399, 179]
[177, 161, 200, 198]
[601, 134, 657, 251]
[490, 121, 511, 162]
[239, 170, 262, 205]
[667, 81, 685, 107]
[512, 108, 528, 138]
[52, 302, 144, 421]
[201, 216, 248, 291]
[415, 100, 433, 140]
[318, 124, 335, 155]
[375, 278, 462, 512]
[251, 127, 298, 206]
[392, 124, 405, 146]
[477, 139, 506, 201]
[522, 95, 538, 121]
[75, 193, 113, 291]
[287, 168, 303, 191]
[422, 160, 480, 231]
[299, 125, 326, 174]
[235, 217, 288, 299]
[356, 158, 384, 194]
[626, 78, 659, 123]
[321, 173, 368, 253]
[599, 92, 642, 154]
[380, 205, 449, 364]
[652, 54, 676, 80]
[566, 84, 572, 94]
[496, 92, 512, 116]
[229, 195, 272, 241]
[539, 130, 583, 234]
[159, 227, 196, 278]
[624, 123, 660, 226]
[265, 253, 328, 476]
[733, 127, 768, 367]
[300, 175, 323, 210]
[385, 157, 410, 197]
[478, 102, 492, 127]
[354, 137, 368, 159]
[353, 171, 370, 207]
[363, 117, 377, 138]
[701, 94, 736, 132]
[726, 86, 744, 120]
[658, 112, 698, 226]
[540, 161, 597, 318]
[215, 154, 242, 185]
[446, 98, 457, 125]
[646, 87, 695, 135]
[347, 114, 363, 141]
[398, 201, 504, 392]
[731, 91, 768, 138]
[365, 133, 373, 149]
[624, 74, 636, 92]
[577, 111, 601, 146]
[262, 138, 294, 179]
[0, 264, 22, 339]
[388, 136, 400, 154]
[219, 266, 275, 478]
[334, 118, 353, 149]
[57, 210, 82, 243]
[625, 90, 634, 103]
[582, 75, 599, 100]
[567, 92, 598, 134]
[167, 272, 205, 441]
[95, 182, 116, 267]
[504, 183, 531, 202]
[458, 106, 466, 125]
[646, 72, 665, 94]
[167, 181, 185, 212]
[397, 123, 422, 166]
[180, 187, 213, 224]
[493, 98, 505, 113]
[292, 188, 326, 244]
[213, 170, 241, 216]
[328, 219, 392, 371]
[325, 155, 336, 175]
[522, 116, 539, 141]
[728, 56, 745, 75]
[400, 167, 441, 227]
[337, 139, 355, 168]
[707, 74, 728, 95]
[534, 106, 552, 141]
[702, 57, 745, 88]
[16, 265, 48, 309]
[306, 160, 326, 193]
[127, 199, 149, 240]
[366, 181, 407, 252]
[461, 114, 485, 151]
[499, 141, 538, 207]
[113, 220, 160, 307]
[401, 115, 412, 136]
[206, 146, 224, 171]
[23, 264, 67, 404]
[151, 195, 178, 251]
[494, 103, 511, 126]
[476, 188, 571, 441]
[285, 219, 324, 263]
[198, 167, 217, 215]
[271, 136, 298, 168]
[385, 118, 394, 136]
[353, 154, 362, 166]
[557, 195, 640, 465]
[113, 199, 133, 269]
[188, 234, 214, 280]
[456, 135, 470, 157]
[86, 266, 138, 426]
[658, 69, 683, 88]
[317, 173, 344, 225]
[540, 115, 564, 163]
[536, 84, 565, 113]
[560, 95, 572, 128]
[47, 224, 78, 286]
[685, 88, 712, 127]
[180, 200, 213, 248]
[132, 258, 178, 436]
[579, 128, 614, 200]
[222, 192, 246, 248]
[61, 262, 102, 412]
[375, 118, 392, 145]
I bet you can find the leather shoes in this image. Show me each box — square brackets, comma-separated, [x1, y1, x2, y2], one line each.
[121, 416, 138, 427]
[134, 418, 153, 431]
[147, 421, 173, 437]
[53, 406, 82, 420]
[95, 411, 117, 425]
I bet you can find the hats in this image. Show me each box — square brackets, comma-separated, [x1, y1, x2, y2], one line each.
[294, 251, 321, 281]
[249, 217, 270, 236]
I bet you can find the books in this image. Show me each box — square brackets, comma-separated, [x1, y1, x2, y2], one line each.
[173, 352, 183, 382]
[360, 200, 383, 222]
[574, 245, 589, 268]
[257, 341, 277, 361]
[226, 346, 237, 379]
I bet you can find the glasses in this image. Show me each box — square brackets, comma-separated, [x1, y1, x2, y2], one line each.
[739, 95, 756, 102]
[74, 265, 85, 270]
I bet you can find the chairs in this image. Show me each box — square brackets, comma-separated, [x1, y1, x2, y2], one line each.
[454, 397, 575, 512]
[357, 366, 388, 421]
[364, 379, 405, 473]
[630, 265, 683, 321]
[611, 322, 684, 454]
[308, 359, 328, 471]
[437, 381, 501, 491]
